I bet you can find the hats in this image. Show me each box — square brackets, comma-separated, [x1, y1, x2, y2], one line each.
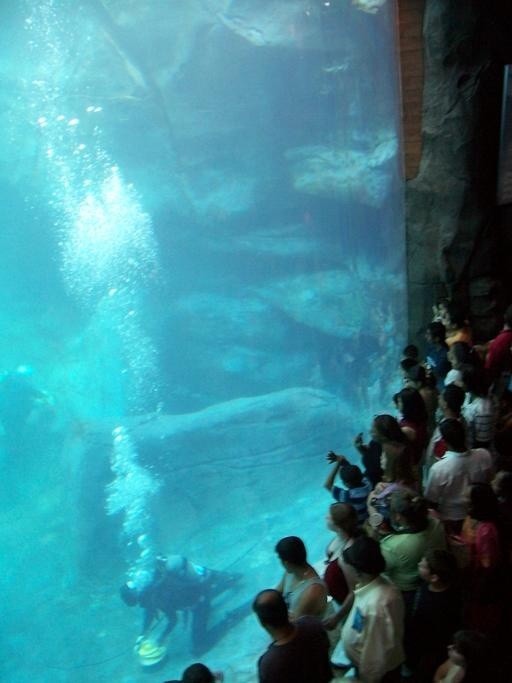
[342, 537, 386, 574]
[119, 583, 140, 606]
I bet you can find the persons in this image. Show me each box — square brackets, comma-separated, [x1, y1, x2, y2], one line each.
[119, 571, 256, 659]
[251, 265, 512, 683]
[180, 663, 224, 683]
